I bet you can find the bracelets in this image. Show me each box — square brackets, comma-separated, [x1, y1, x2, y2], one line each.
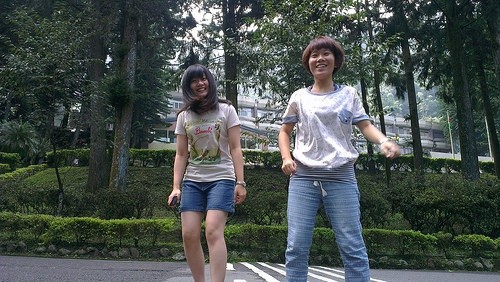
[379, 136, 391, 145]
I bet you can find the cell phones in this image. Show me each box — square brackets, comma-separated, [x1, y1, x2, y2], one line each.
[170, 197, 177, 206]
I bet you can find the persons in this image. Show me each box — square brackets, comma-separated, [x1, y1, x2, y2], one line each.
[278, 37, 401, 282]
[167, 65, 248, 282]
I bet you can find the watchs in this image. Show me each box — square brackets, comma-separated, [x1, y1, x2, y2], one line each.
[236, 182, 246, 187]
